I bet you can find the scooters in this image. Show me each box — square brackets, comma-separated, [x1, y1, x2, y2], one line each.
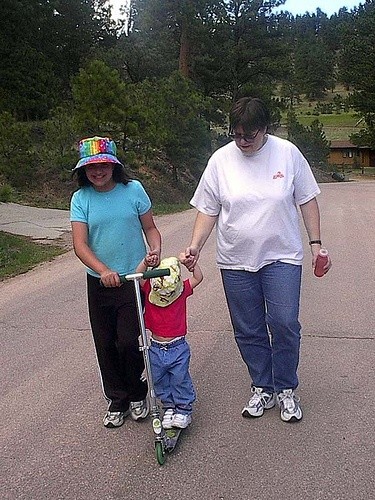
[100, 267, 185, 466]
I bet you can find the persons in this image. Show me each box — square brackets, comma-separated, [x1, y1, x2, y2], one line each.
[136, 252, 203, 428]
[185, 97, 332, 423]
[71, 138, 161, 428]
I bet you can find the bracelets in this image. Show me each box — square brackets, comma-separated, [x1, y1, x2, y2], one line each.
[309, 240, 322, 246]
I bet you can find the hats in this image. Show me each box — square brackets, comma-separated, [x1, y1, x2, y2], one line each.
[72, 137, 125, 172]
[148, 256, 183, 307]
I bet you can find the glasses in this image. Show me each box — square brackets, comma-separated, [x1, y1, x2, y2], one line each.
[228, 127, 261, 142]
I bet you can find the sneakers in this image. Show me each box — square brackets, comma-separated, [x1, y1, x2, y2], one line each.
[171, 412, 192, 428]
[241, 386, 275, 418]
[130, 394, 149, 421]
[276, 388, 302, 422]
[103, 407, 130, 427]
[162, 409, 172, 429]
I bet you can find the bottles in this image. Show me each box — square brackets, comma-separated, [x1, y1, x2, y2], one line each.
[314, 247, 328, 278]
